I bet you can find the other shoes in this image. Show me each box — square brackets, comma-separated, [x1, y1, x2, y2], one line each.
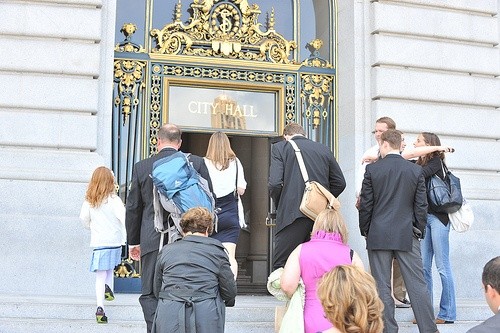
[413, 317, 454, 324]
[393, 296, 411, 308]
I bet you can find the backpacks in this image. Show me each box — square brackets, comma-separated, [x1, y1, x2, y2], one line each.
[151, 151, 215, 237]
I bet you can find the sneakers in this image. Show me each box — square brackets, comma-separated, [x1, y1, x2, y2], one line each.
[105, 284, 114, 301]
[96, 306, 107, 323]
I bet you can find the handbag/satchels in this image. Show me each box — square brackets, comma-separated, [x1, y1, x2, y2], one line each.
[300, 181, 341, 222]
[427, 152, 463, 213]
[237, 194, 247, 229]
[437, 155, 474, 233]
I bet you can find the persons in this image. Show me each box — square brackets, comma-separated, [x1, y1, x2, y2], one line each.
[150, 206, 237, 333]
[203, 130, 247, 286]
[126, 125, 217, 333]
[269, 121, 346, 269]
[80, 167, 125, 323]
[356, 116, 456, 333]
[281, 211, 385, 333]
[464, 257, 500, 333]
